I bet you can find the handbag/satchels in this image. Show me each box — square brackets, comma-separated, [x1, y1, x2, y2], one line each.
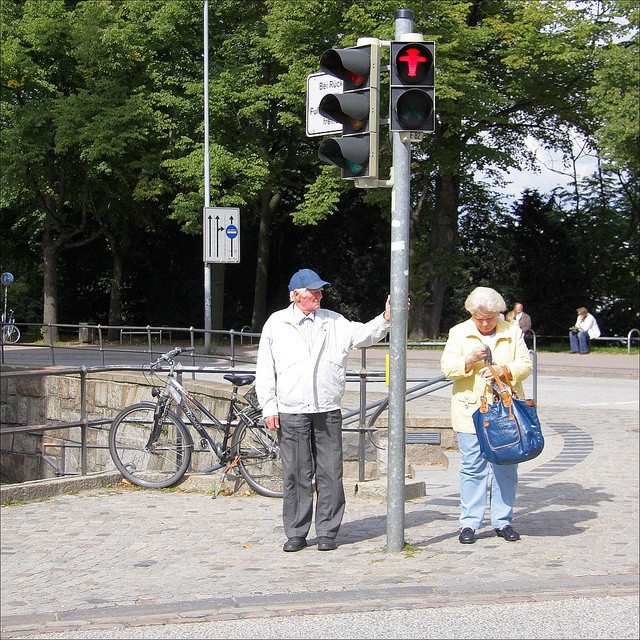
[472, 366, 544, 465]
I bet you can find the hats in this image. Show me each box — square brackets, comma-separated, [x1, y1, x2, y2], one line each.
[288, 268, 331, 291]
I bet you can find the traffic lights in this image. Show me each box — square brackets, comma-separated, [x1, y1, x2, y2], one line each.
[390, 42, 435, 132]
[319, 44, 379, 179]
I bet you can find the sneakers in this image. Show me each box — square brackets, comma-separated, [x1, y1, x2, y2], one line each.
[495, 525, 519, 540]
[459, 527, 475, 543]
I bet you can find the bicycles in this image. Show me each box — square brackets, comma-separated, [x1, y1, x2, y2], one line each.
[108, 346, 315, 498]
[2, 309, 21, 344]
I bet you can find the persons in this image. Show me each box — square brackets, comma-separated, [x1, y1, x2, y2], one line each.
[505, 301, 532, 339]
[567, 307, 602, 354]
[254, 267, 410, 551]
[439, 286, 534, 546]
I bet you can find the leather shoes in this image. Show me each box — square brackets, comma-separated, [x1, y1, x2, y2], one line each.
[318, 536, 336, 549]
[283, 537, 307, 551]
